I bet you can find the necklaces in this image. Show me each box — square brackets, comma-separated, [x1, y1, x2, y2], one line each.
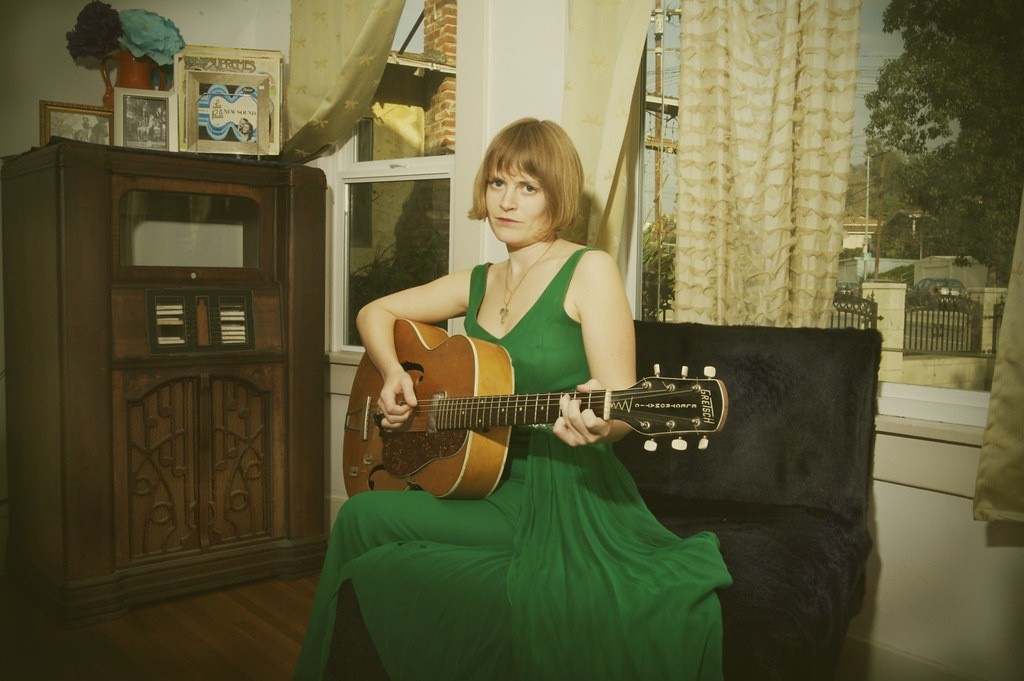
[500, 235, 558, 322]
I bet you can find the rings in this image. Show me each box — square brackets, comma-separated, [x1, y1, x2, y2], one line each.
[374, 413, 386, 432]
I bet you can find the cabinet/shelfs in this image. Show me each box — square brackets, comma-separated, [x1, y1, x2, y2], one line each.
[0, 137, 331, 623]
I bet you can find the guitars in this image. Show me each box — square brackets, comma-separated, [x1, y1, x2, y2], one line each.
[343, 316, 729, 501]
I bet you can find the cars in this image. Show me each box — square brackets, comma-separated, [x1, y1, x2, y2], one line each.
[834, 281, 862, 298]
[906, 278, 972, 313]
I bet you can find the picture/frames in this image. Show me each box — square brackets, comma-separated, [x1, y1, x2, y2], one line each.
[113, 86, 178, 152]
[39, 100, 113, 147]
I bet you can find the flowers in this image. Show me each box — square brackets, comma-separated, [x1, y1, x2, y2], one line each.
[65, 0, 186, 91]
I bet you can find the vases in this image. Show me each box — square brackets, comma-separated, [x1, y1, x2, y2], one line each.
[99, 48, 168, 111]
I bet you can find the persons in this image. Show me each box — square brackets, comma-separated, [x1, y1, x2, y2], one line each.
[290, 117, 734, 681]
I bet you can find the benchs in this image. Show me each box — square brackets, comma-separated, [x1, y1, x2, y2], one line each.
[610, 320, 884, 681]
